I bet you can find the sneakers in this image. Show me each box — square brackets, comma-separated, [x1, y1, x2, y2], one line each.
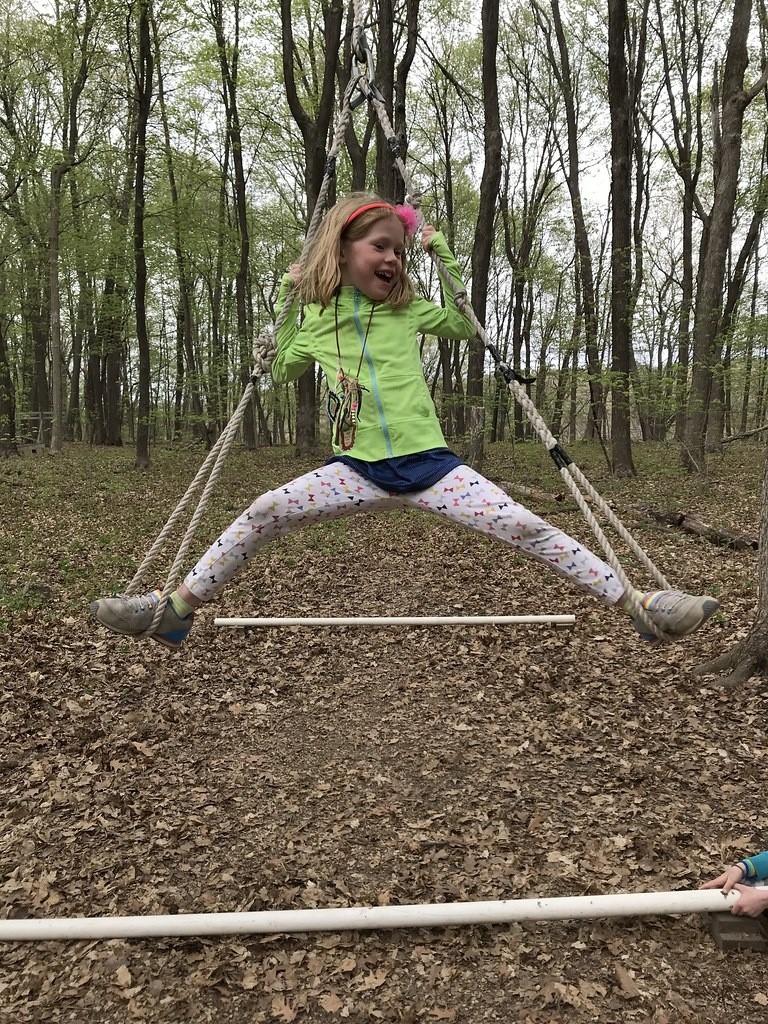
[632, 591, 720, 637]
[90, 589, 198, 650]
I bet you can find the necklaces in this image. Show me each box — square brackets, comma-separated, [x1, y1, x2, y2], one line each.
[334, 286, 375, 425]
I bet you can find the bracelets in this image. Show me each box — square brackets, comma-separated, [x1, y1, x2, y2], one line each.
[733, 864, 745, 877]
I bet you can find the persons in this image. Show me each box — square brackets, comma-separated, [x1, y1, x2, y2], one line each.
[699, 851, 768, 918]
[90, 196, 718, 648]
